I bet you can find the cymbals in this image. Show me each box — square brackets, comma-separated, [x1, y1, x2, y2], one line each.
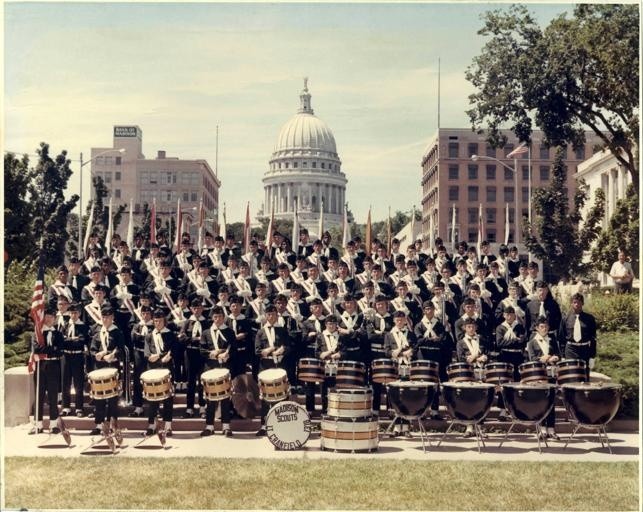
[231, 372, 259, 419]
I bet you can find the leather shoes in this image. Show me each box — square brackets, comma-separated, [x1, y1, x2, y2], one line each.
[28, 427, 43, 435]
[222, 429, 232, 437]
[88, 411, 96, 418]
[75, 410, 84, 417]
[292, 385, 398, 420]
[140, 428, 157, 435]
[388, 413, 569, 439]
[200, 429, 214, 436]
[128, 410, 145, 417]
[90, 428, 101, 435]
[163, 427, 172, 436]
[59, 408, 73, 416]
[256, 428, 267, 435]
[153, 402, 260, 419]
[49, 427, 60, 433]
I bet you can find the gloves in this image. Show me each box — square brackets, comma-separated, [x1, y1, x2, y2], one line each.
[588, 358, 595, 370]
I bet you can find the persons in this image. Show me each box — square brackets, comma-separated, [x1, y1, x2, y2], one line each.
[29, 234, 597, 439]
[608, 251, 634, 294]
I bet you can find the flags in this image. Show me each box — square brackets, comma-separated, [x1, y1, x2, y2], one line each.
[317, 209, 326, 240]
[83, 203, 95, 255]
[173, 205, 185, 249]
[476, 214, 485, 258]
[240, 205, 252, 243]
[386, 217, 393, 254]
[342, 215, 352, 248]
[126, 208, 136, 253]
[264, 212, 274, 257]
[149, 205, 159, 254]
[365, 211, 374, 257]
[196, 206, 207, 250]
[291, 214, 300, 254]
[103, 205, 113, 255]
[507, 142, 530, 160]
[219, 209, 227, 239]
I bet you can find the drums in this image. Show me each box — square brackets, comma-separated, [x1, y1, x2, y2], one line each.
[265, 401, 311, 450]
[320, 389, 380, 452]
[297, 357, 592, 388]
[140, 368, 175, 402]
[201, 367, 233, 400]
[387, 381, 621, 429]
[258, 367, 291, 402]
[87, 367, 123, 399]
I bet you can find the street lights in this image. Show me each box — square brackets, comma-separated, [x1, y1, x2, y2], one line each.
[75, 143, 128, 258]
[469, 152, 522, 253]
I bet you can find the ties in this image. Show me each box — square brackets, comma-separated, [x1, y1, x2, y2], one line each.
[574, 314, 581, 343]
